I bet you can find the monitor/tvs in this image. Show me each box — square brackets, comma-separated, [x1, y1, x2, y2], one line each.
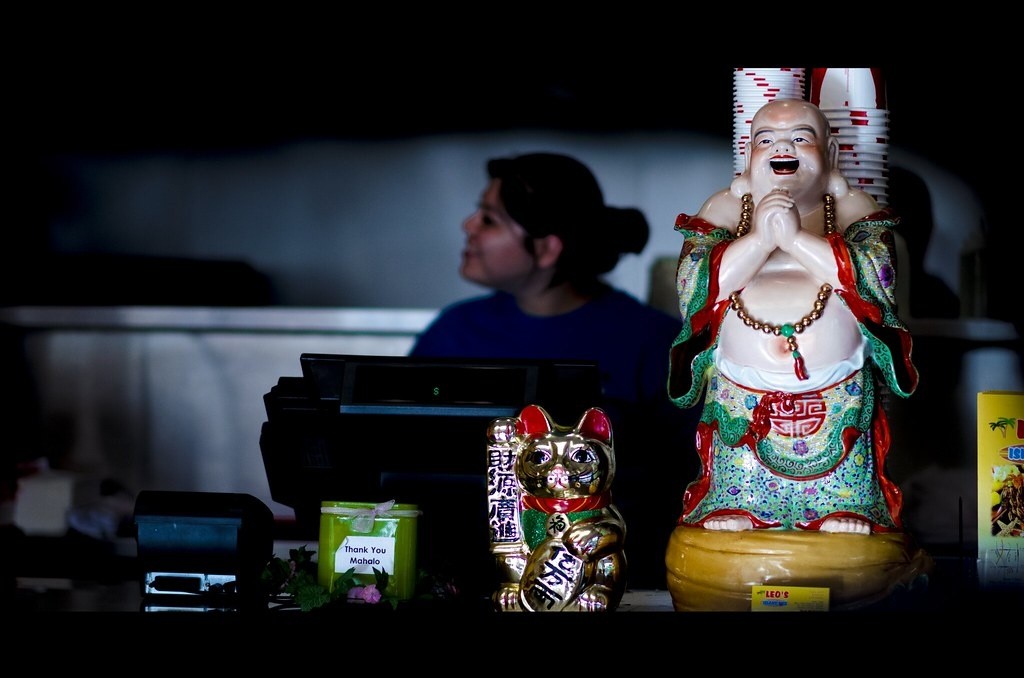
[300, 353, 605, 602]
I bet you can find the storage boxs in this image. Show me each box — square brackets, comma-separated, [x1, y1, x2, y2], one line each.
[975, 388, 1024, 584]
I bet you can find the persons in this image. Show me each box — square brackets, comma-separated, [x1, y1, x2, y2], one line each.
[410, 152, 682, 406]
[666, 100, 920, 535]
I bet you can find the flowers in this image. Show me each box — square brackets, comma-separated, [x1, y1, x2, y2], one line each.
[260, 541, 462, 612]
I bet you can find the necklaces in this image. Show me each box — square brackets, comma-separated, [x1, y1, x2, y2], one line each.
[729, 195, 837, 382]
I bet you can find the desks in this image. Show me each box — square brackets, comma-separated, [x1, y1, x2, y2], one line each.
[0, 537, 1024, 678]
